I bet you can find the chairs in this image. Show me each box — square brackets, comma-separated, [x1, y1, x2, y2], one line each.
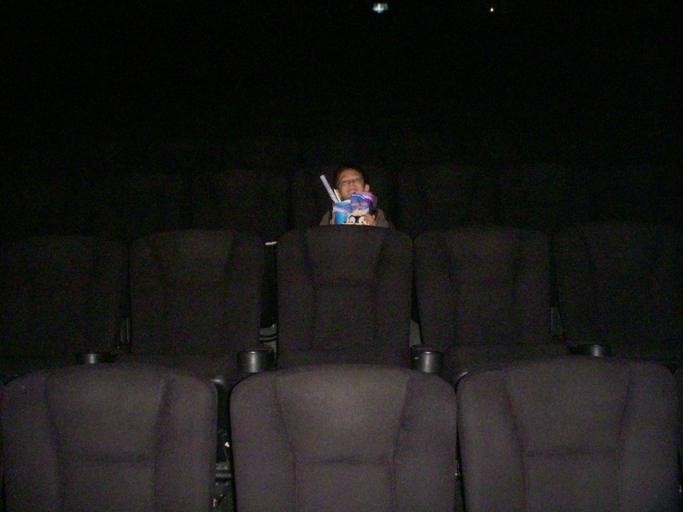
[457, 354, 681, 510]
[228, 362, 458, 510]
[2, 362, 219, 511]
[1, 165, 682, 480]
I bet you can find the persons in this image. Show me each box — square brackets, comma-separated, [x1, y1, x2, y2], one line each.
[319, 164, 389, 229]
